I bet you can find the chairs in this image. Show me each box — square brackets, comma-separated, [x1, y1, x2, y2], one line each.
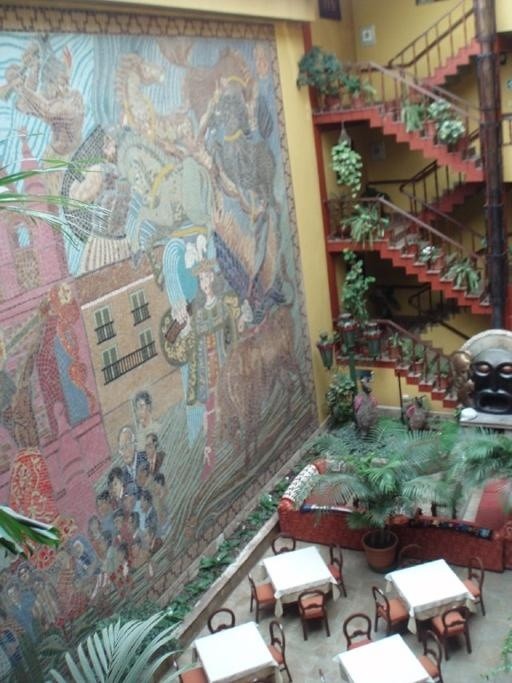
[272, 533, 296, 555]
[463, 556, 485, 616]
[208, 608, 235, 633]
[172, 655, 208, 683]
[248, 572, 276, 624]
[266, 620, 293, 682]
[418, 630, 443, 683]
[327, 542, 347, 597]
[372, 585, 409, 637]
[343, 613, 373, 651]
[298, 590, 330, 640]
[431, 608, 471, 660]
[399, 544, 425, 568]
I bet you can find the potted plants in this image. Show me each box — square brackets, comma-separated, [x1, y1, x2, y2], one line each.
[406, 344, 427, 373]
[388, 332, 402, 358]
[338, 203, 389, 243]
[400, 98, 432, 134]
[437, 120, 466, 152]
[418, 245, 445, 270]
[434, 361, 452, 388]
[405, 238, 420, 254]
[296, 45, 349, 111]
[305, 419, 466, 572]
[344, 75, 376, 108]
